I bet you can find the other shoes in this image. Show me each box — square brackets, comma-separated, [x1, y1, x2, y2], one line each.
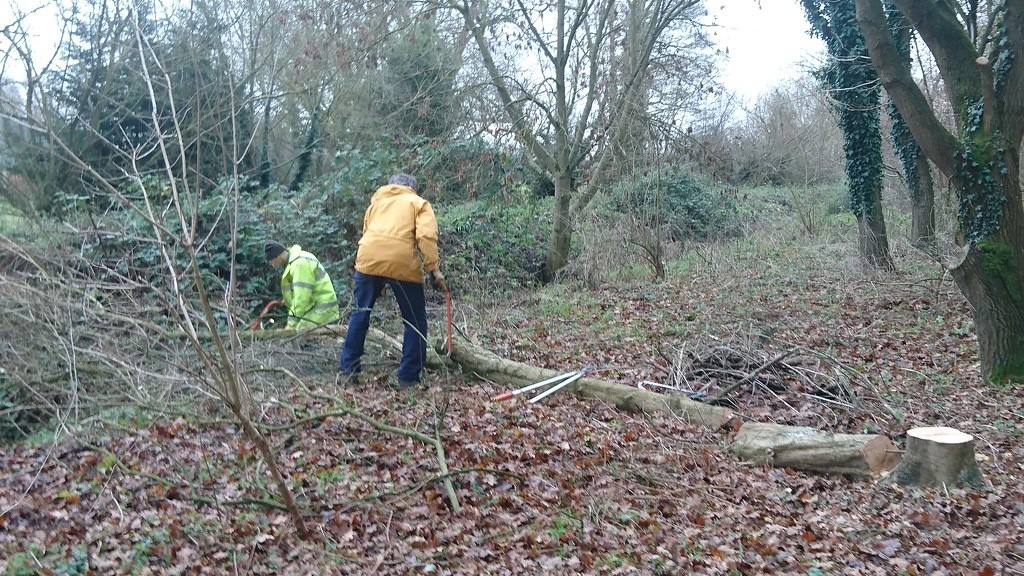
[400, 379, 419, 388]
[337, 373, 359, 386]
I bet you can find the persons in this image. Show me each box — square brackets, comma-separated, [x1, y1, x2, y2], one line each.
[339, 173, 447, 387]
[264, 241, 339, 332]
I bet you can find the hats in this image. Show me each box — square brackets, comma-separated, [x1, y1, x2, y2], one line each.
[265, 239, 286, 261]
